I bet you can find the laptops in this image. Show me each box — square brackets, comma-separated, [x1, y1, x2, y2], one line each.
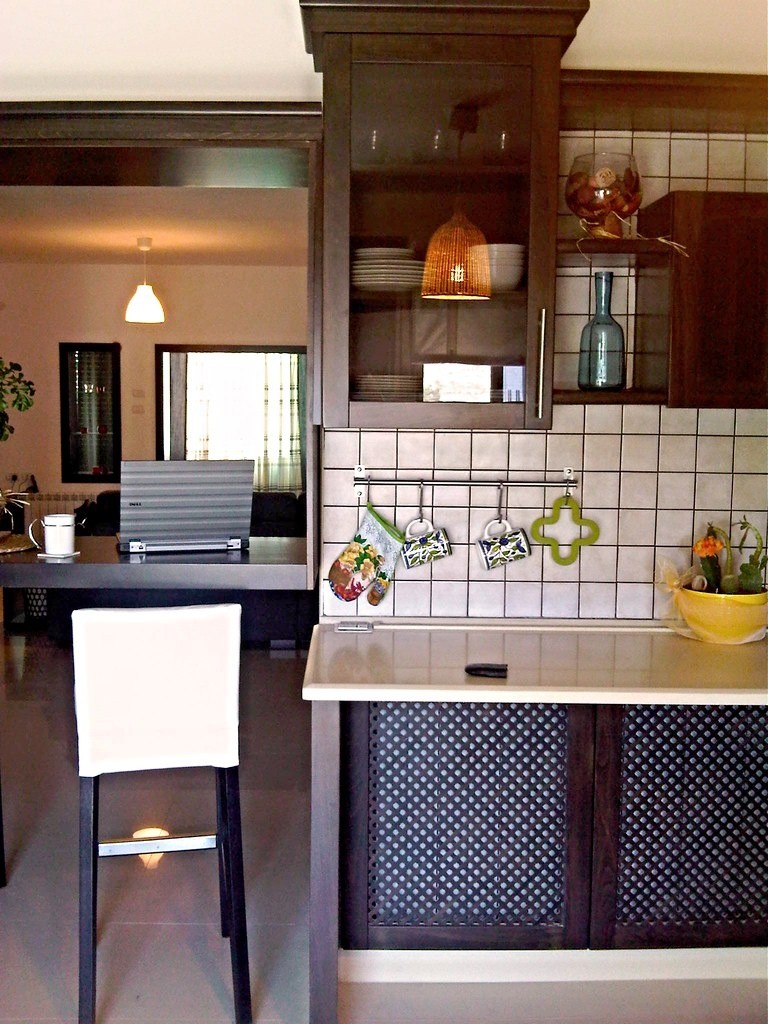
[117, 459, 255, 555]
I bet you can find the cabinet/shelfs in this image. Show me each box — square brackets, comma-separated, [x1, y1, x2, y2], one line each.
[296, 0, 768, 433]
[310, 704, 768, 1024]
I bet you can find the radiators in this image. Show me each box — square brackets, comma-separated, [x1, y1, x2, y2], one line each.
[23, 492, 95, 537]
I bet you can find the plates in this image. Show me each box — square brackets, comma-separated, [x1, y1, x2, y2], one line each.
[351, 248, 426, 290]
[353, 375, 504, 402]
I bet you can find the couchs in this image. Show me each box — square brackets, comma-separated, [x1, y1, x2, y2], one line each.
[42, 489, 308, 650]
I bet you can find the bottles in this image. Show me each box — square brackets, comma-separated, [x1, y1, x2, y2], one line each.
[578, 272, 625, 391]
[564, 152, 642, 239]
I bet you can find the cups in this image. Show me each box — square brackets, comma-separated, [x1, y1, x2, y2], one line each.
[475, 519, 532, 571]
[43, 515, 75, 555]
[400, 518, 452, 570]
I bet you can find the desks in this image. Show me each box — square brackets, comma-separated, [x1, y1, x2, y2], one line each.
[0, 535, 307, 591]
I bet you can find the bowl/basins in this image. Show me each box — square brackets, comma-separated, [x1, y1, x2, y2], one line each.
[467, 244, 525, 290]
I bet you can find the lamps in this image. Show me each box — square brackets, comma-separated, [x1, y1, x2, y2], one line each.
[420, 102, 491, 301]
[123, 237, 166, 323]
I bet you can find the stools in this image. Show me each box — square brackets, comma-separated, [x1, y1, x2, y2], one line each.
[72, 602, 253, 1024]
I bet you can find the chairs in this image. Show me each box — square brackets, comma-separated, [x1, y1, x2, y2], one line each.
[0, 470, 37, 493]
